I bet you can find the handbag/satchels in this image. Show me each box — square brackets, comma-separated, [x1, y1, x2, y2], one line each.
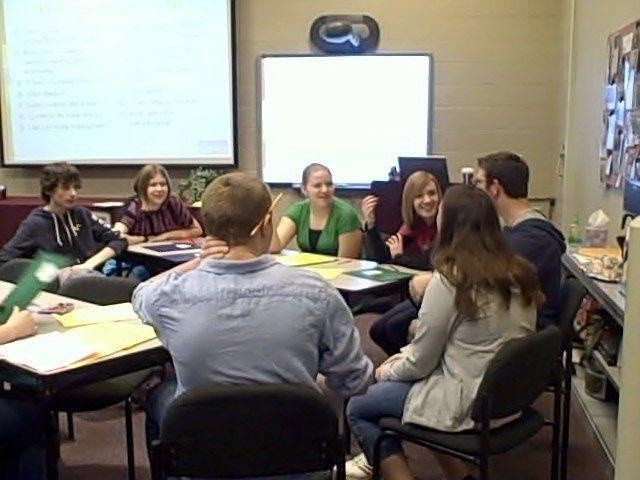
[572, 296, 623, 401]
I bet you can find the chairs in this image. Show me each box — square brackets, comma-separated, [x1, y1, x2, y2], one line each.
[146, 372, 348, 479]
[371, 275, 586, 478]
[0, 258, 167, 480]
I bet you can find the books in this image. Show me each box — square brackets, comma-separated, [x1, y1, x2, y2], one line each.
[1, 247, 69, 323]
[277, 253, 340, 269]
[344, 267, 419, 282]
[145, 243, 200, 252]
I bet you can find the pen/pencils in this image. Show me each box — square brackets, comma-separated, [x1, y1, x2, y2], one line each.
[389, 264, 399, 273]
[247, 194, 285, 238]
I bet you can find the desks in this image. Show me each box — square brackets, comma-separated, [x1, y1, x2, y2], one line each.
[116, 236, 424, 458]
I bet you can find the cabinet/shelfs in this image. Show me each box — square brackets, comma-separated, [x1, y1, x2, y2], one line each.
[560, 243, 626, 469]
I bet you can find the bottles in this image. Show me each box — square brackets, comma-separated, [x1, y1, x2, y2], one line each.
[569, 214, 581, 247]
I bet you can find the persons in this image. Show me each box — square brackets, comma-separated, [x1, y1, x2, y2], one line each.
[128, 171, 376, 480]
[357, 170, 446, 357]
[0, 161, 130, 291]
[103, 163, 203, 281]
[332, 150, 568, 480]
[343, 185, 549, 480]
[0, 302, 63, 480]
[264, 163, 364, 266]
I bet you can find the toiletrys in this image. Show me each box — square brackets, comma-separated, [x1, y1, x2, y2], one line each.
[568, 213, 584, 247]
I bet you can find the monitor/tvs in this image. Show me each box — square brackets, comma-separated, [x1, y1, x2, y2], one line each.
[397, 155, 449, 187]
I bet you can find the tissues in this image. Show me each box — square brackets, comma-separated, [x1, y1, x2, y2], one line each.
[584, 209, 610, 246]
